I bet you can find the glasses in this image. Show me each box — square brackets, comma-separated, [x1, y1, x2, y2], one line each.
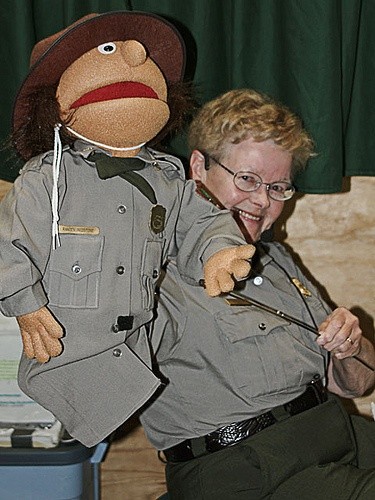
[202, 150, 295, 201]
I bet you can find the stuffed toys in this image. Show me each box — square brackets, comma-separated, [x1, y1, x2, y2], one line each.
[1, 11, 256, 448]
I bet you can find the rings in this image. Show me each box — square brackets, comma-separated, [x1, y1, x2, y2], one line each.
[347, 336, 355, 346]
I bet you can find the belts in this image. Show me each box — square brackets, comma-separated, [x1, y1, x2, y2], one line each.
[162, 383, 329, 464]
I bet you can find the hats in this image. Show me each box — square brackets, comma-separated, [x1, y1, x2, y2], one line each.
[11, 10, 187, 161]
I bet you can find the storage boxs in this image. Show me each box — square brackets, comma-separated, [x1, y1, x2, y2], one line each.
[0, 422, 109, 500]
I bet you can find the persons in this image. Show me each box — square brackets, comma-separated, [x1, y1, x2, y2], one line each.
[138, 88, 375, 500]
